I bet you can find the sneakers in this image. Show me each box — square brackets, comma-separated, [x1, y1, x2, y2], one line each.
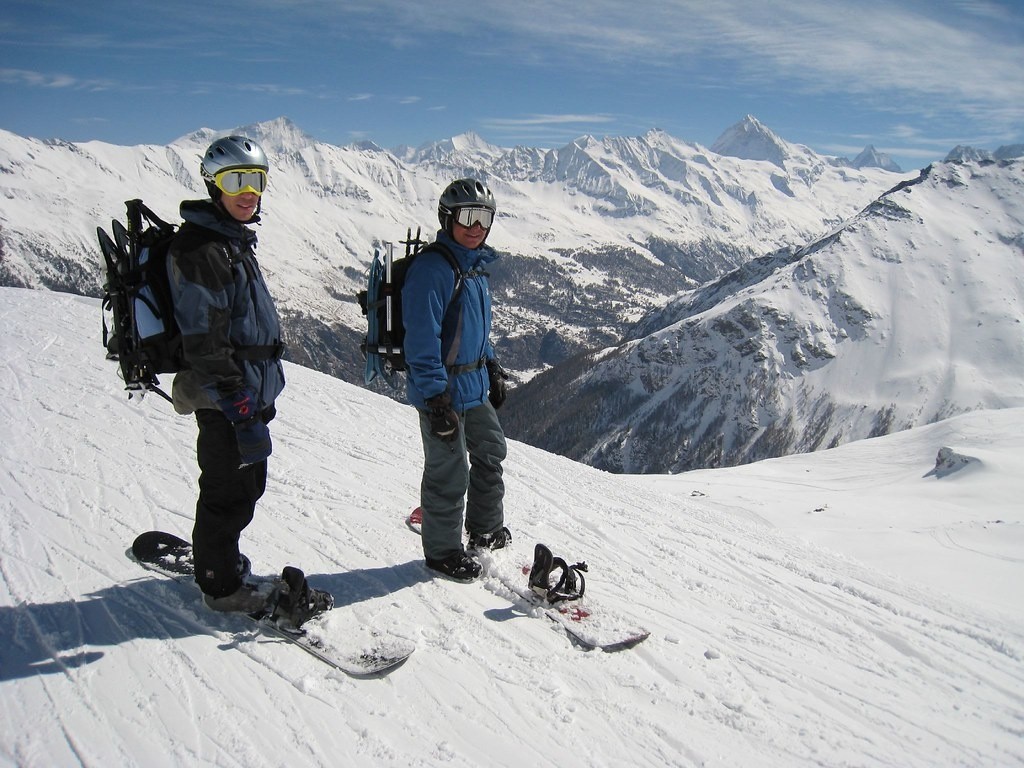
[425, 543, 483, 584]
[466, 526, 512, 551]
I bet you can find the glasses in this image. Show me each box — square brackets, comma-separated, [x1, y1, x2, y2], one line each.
[438, 205, 494, 228]
[200, 163, 268, 196]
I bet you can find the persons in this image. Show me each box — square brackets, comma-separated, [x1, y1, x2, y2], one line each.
[166, 137, 284, 610]
[400, 178, 510, 584]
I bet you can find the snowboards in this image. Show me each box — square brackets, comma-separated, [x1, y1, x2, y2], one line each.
[406, 502, 648, 647]
[129, 530, 415, 679]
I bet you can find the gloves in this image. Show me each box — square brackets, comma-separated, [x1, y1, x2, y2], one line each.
[485, 359, 509, 410]
[216, 388, 272, 464]
[424, 393, 459, 443]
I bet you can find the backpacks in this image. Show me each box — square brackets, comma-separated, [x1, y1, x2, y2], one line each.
[358, 242, 463, 385]
[96, 199, 235, 405]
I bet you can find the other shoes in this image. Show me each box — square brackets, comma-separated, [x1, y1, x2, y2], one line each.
[203, 587, 268, 612]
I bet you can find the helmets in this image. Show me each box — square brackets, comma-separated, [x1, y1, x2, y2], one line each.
[199, 136, 269, 202]
[437, 178, 496, 232]
